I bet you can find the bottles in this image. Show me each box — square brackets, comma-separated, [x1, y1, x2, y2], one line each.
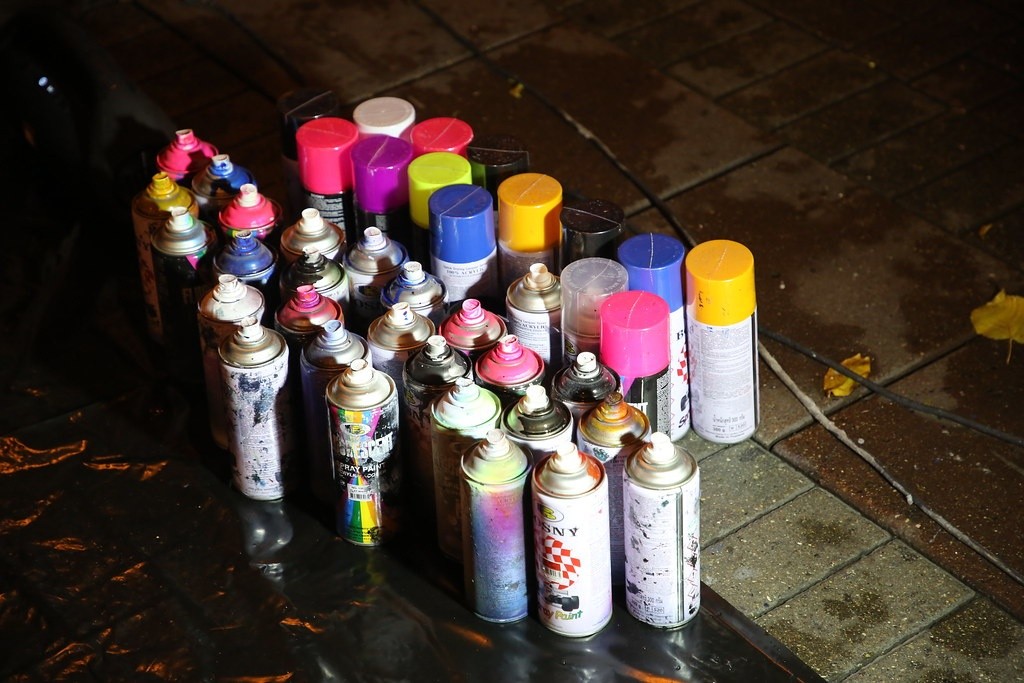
[134, 97, 760, 637]
